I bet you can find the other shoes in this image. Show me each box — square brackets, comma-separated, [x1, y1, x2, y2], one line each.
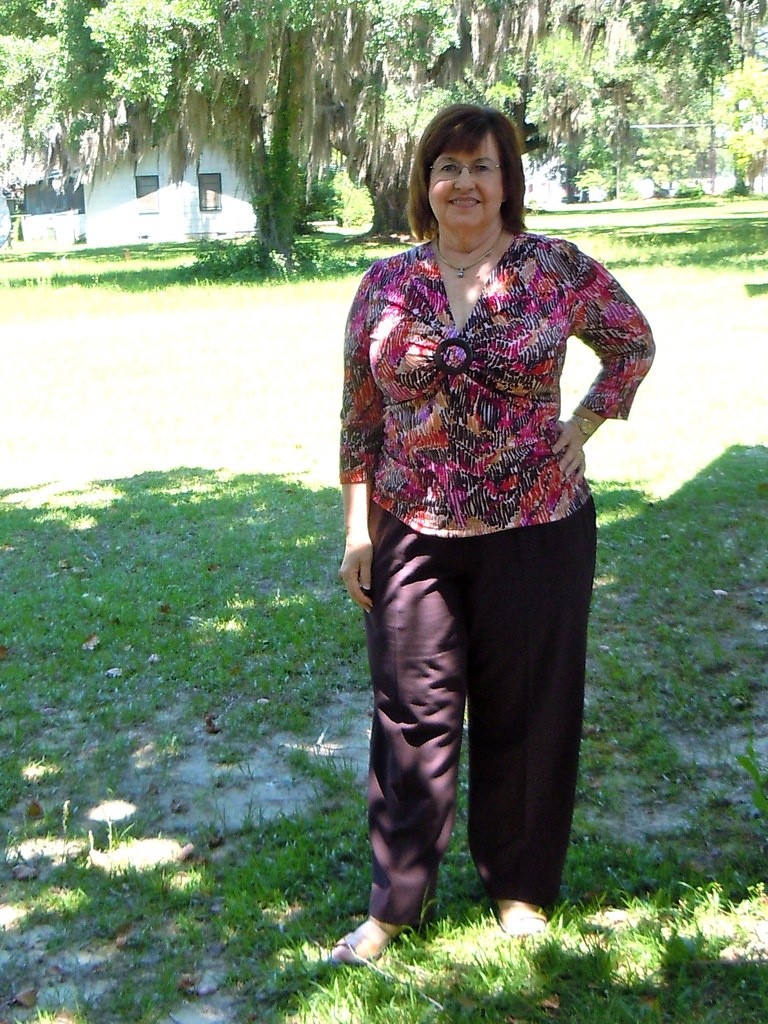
[496, 900, 548, 938]
[333, 915, 408, 964]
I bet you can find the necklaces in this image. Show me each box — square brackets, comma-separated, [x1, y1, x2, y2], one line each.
[437, 228, 503, 277]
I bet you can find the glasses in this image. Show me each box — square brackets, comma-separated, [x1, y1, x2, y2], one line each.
[430, 158, 502, 180]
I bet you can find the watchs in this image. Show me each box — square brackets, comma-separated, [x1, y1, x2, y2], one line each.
[574, 414, 595, 436]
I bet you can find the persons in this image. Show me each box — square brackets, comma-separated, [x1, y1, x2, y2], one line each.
[329, 104, 656, 965]
